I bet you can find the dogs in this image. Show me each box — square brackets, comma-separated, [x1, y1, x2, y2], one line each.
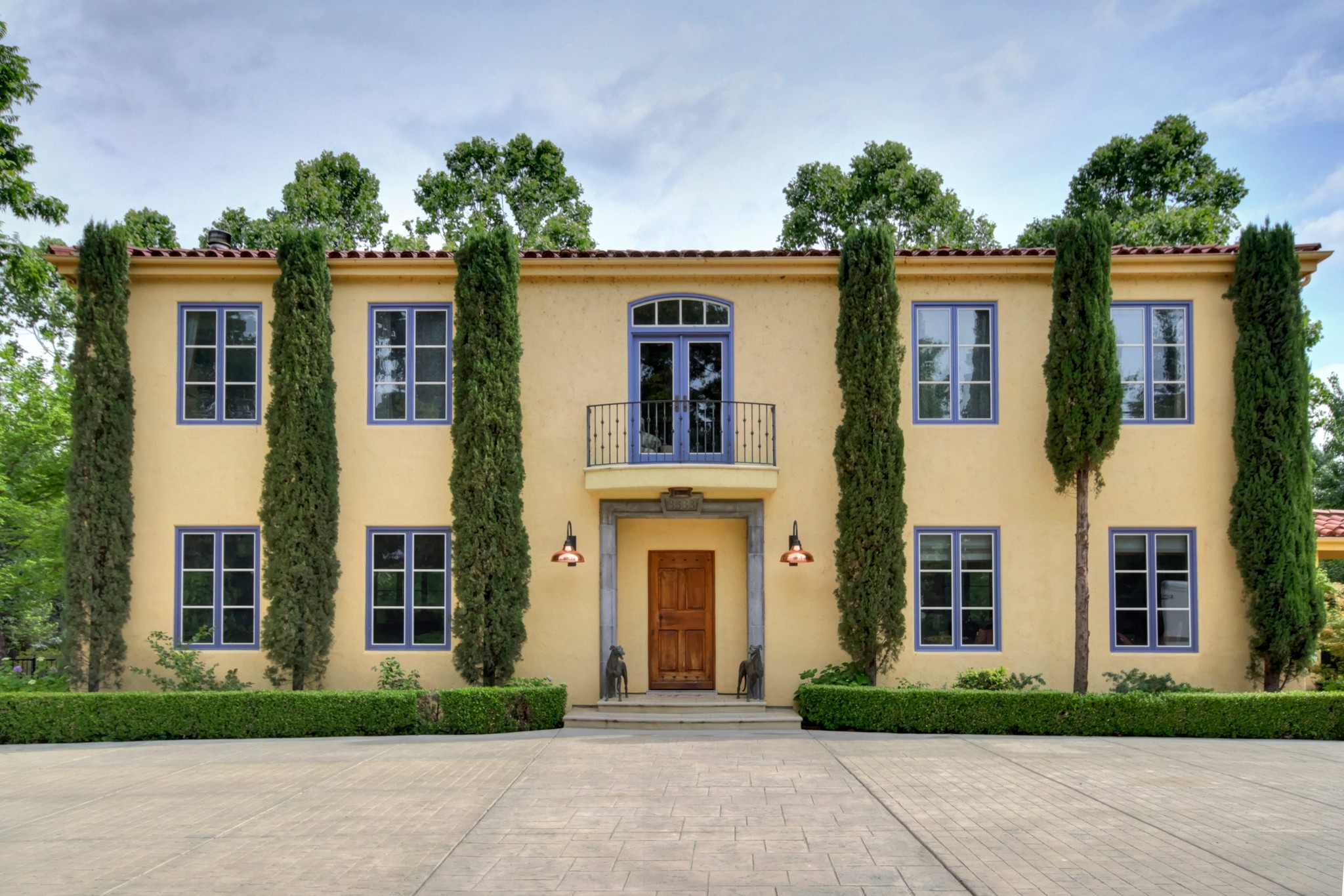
[604, 645, 629, 702]
[736, 644, 764, 702]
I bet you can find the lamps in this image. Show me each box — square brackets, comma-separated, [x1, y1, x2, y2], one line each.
[779, 520, 815, 566]
[550, 520, 584, 568]
